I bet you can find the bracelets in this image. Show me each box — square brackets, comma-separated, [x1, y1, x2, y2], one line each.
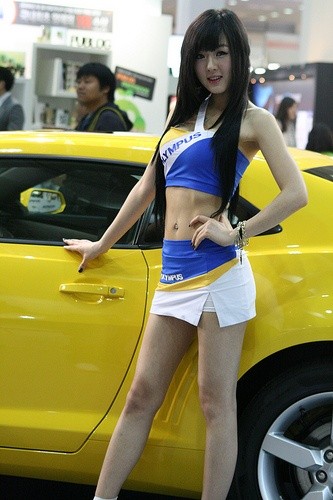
[235, 220, 248, 264]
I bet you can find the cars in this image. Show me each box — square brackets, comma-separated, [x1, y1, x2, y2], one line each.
[1, 129, 332, 500]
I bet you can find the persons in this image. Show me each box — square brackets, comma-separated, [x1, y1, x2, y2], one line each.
[75, 62, 134, 134]
[0, 67, 25, 131]
[275, 97, 298, 148]
[62, 8, 308, 500]
